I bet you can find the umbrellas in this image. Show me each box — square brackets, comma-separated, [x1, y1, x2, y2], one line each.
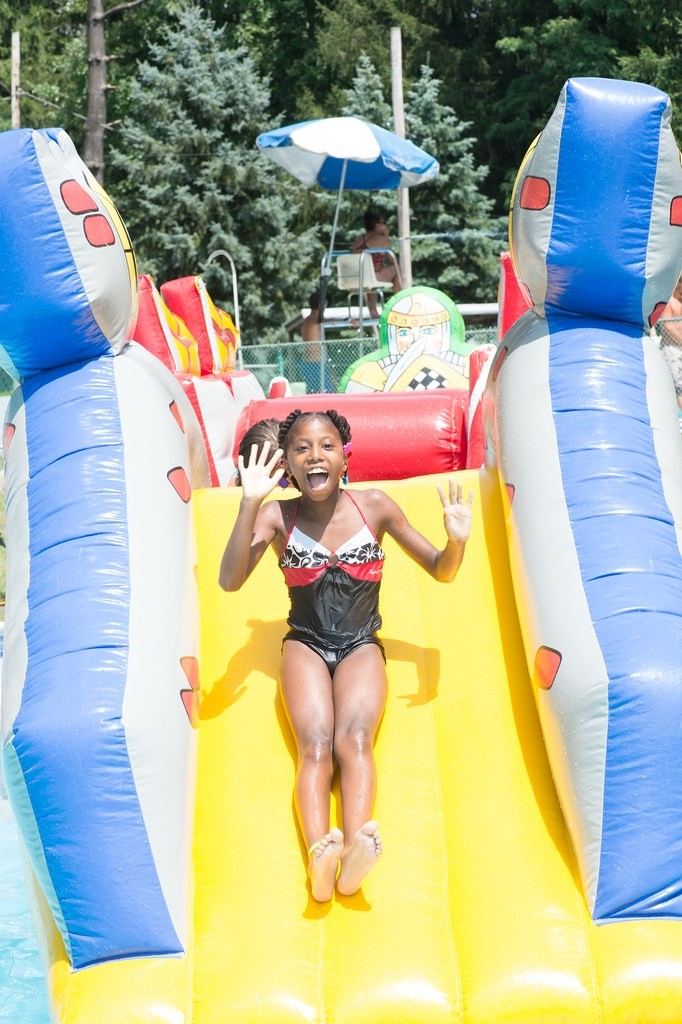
[256, 117, 441, 277]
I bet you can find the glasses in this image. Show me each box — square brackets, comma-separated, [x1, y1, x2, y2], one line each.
[376, 220, 387, 225]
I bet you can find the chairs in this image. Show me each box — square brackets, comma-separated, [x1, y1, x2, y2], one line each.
[338, 253, 393, 321]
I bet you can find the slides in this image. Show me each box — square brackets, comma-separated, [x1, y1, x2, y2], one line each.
[179, 462, 603, 1024]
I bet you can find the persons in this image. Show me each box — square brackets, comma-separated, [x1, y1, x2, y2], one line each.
[660, 278, 682, 409]
[302, 294, 359, 394]
[219, 409, 474, 903]
[352, 211, 403, 318]
[227, 418, 284, 486]
